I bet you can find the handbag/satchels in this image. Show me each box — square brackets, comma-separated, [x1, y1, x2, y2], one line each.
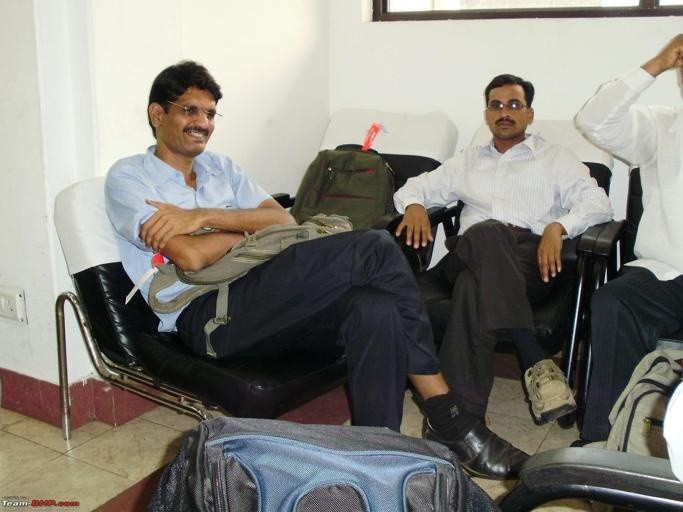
[147, 417, 502, 512]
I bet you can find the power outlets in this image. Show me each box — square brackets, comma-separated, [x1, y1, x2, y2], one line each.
[0, 287, 27, 327]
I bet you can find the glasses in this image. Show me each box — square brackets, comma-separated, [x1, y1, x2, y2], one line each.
[167, 99, 223, 121]
[487, 100, 529, 112]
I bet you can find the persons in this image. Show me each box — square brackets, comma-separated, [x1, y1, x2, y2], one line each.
[104, 62, 530, 480]
[571, 32, 683, 448]
[393, 74, 611, 428]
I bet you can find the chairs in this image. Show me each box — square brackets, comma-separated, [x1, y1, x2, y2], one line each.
[49, 175, 352, 446]
[266, 97, 460, 276]
[492, 337, 682, 512]
[565, 159, 645, 447]
[387, 106, 625, 428]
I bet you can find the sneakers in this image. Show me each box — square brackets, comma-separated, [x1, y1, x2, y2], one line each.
[525, 360, 577, 424]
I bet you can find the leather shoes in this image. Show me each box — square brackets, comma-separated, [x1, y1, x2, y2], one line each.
[424, 417, 531, 479]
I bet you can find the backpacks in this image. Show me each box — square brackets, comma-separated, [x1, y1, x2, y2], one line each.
[146, 215, 350, 313]
[608, 349, 683, 455]
[291, 144, 393, 230]
